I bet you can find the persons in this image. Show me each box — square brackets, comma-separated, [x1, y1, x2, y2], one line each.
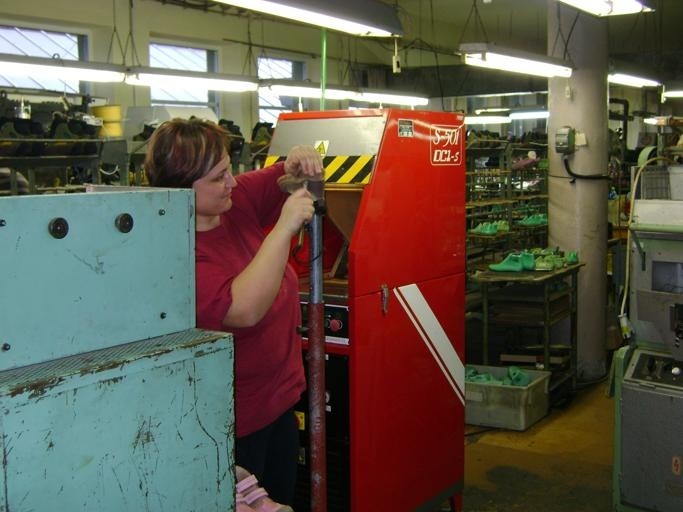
[149, 116, 324, 496]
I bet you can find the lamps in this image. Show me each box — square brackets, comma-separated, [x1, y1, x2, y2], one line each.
[608, 34, 662, 89]
[344, 38, 430, 105]
[1, 0, 128, 84]
[254, 20, 360, 103]
[122, 0, 258, 93]
[221, 0, 404, 40]
[564, 1, 653, 17]
[455, 2, 577, 77]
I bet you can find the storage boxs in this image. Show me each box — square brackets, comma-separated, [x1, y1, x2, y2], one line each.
[464, 364, 552, 431]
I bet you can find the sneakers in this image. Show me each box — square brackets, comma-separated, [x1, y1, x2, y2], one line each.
[488, 246, 578, 272]
[235, 464, 294, 511]
[468, 220, 509, 236]
[517, 213, 548, 227]
[465, 128, 547, 147]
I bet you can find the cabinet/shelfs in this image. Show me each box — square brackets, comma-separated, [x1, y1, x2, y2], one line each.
[458, 134, 586, 403]
